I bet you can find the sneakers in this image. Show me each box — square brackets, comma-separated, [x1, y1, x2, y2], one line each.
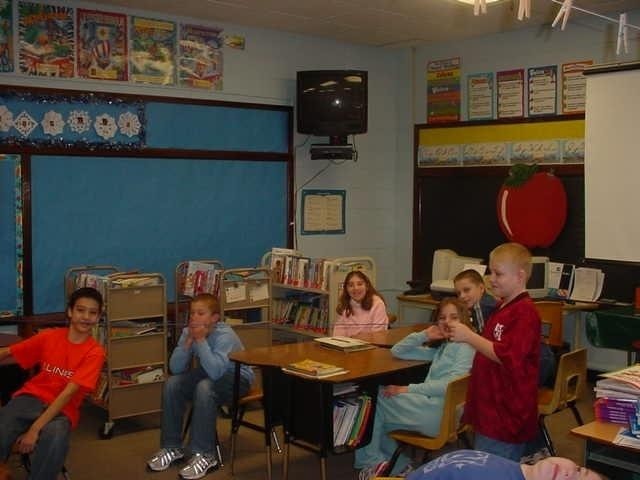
[146, 447, 185, 471]
[359, 461, 389, 480]
[177, 454, 218, 480]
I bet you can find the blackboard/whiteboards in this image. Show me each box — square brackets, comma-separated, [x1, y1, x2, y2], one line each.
[412, 164, 640, 304]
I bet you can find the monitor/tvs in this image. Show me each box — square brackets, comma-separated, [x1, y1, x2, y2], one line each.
[525, 256, 549, 299]
[295, 69, 369, 159]
[430, 249, 485, 294]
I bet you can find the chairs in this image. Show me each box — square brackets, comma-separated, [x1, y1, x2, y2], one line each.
[177, 327, 271, 475]
[379, 373, 472, 479]
[533, 301, 563, 346]
[535, 347, 589, 457]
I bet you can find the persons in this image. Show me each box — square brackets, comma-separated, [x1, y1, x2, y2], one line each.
[442, 243, 542, 463]
[0, 287, 108, 480]
[146, 292, 253, 479]
[354, 296, 476, 477]
[404, 449, 607, 480]
[332, 271, 389, 337]
[453, 267, 499, 336]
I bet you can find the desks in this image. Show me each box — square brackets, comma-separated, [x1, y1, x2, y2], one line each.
[0, 332, 27, 366]
[570, 418, 640, 480]
[227, 323, 434, 480]
[397, 294, 600, 350]
[586, 305, 640, 367]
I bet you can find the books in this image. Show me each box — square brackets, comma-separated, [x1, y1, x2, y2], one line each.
[90, 320, 158, 347]
[92, 365, 163, 410]
[313, 334, 376, 353]
[281, 357, 350, 381]
[66, 269, 160, 314]
[178, 260, 223, 300]
[332, 381, 372, 449]
[269, 247, 366, 337]
[593, 362, 640, 450]
